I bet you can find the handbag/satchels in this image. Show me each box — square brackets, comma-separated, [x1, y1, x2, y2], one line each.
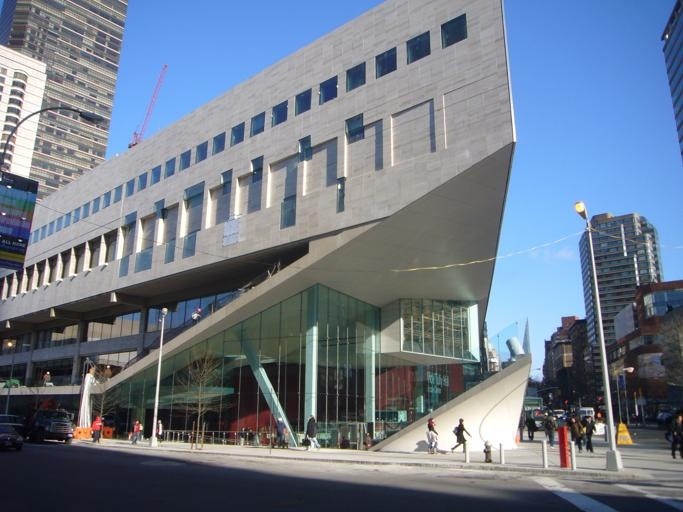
[302, 436, 311, 446]
[545, 421, 553, 430]
[452, 427, 458, 435]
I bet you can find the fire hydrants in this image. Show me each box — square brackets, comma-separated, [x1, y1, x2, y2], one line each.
[483, 442, 495, 463]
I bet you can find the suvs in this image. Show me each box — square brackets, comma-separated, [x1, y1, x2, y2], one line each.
[0, 411, 25, 439]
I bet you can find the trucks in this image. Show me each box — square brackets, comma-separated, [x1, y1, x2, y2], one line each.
[0, 395, 75, 446]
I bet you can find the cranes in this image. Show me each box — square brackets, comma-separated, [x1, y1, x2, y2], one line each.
[126, 60, 173, 154]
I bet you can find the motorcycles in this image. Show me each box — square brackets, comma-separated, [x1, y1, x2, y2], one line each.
[657, 410, 683, 442]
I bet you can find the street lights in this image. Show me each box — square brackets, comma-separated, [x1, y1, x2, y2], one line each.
[5, 341, 16, 416]
[572, 389, 582, 406]
[0, 106, 104, 169]
[148, 305, 168, 447]
[615, 365, 637, 425]
[570, 196, 621, 470]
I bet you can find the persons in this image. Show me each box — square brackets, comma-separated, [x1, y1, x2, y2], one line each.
[363, 432, 373, 450]
[238, 427, 256, 446]
[158, 420, 163, 441]
[90, 364, 113, 383]
[339, 436, 351, 449]
[304, 415, 320, 450]
[90, 415, 102, 443]
[43, 371, 51, 386]
[274, 417, 286, 448]
[671, 414, 683, 459]
[131, 419, 140, 445]
[450, 418, 471, 453]
[427, 418, 439, 454]
[524, 412, 597, 453]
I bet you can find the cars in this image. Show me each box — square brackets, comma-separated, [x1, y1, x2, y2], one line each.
[0, 425, 24, 450]
[312, 405, 406, 447]
[534, 405, 596, 431]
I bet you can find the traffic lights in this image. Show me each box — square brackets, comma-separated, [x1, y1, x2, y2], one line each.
[562, 398, 568, 409]
[595, 410, 606, 418]
[596, 393, 602, 401]
